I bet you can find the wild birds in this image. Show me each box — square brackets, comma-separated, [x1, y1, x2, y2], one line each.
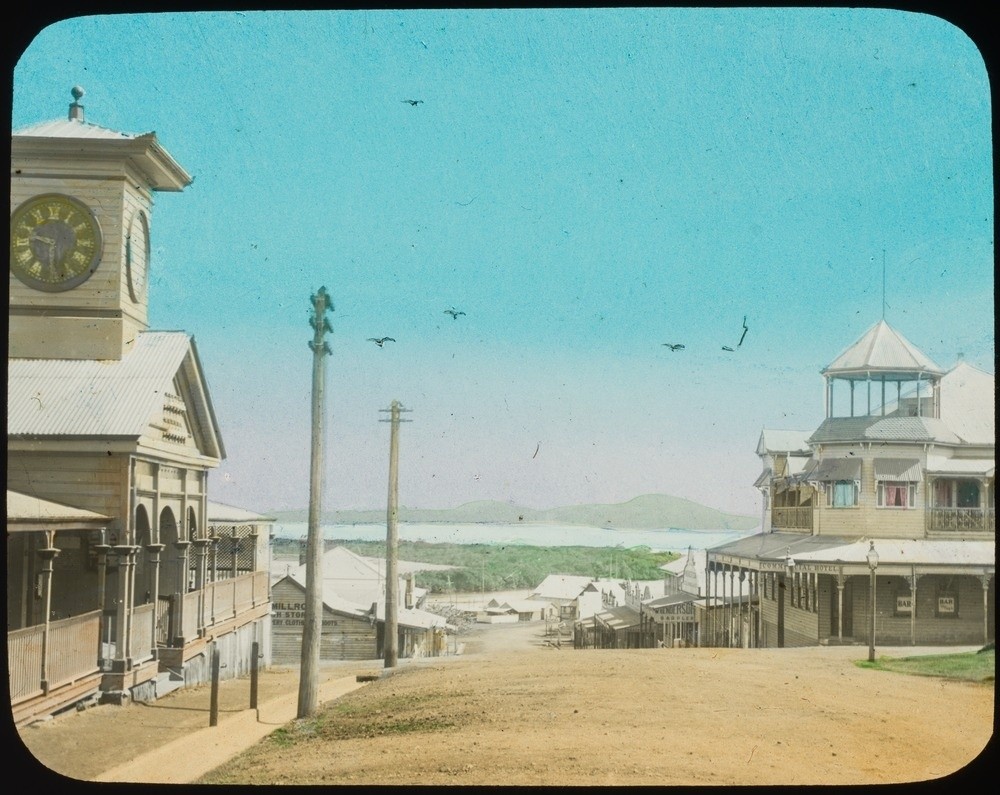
[660, 342, 686, 352]
[444, 309, 467, 320]
[365, 337, 396, 348]
[400, 100, 425, 106]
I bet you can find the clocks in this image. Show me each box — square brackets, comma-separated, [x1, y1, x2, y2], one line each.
[10, 193, 103, 293]
